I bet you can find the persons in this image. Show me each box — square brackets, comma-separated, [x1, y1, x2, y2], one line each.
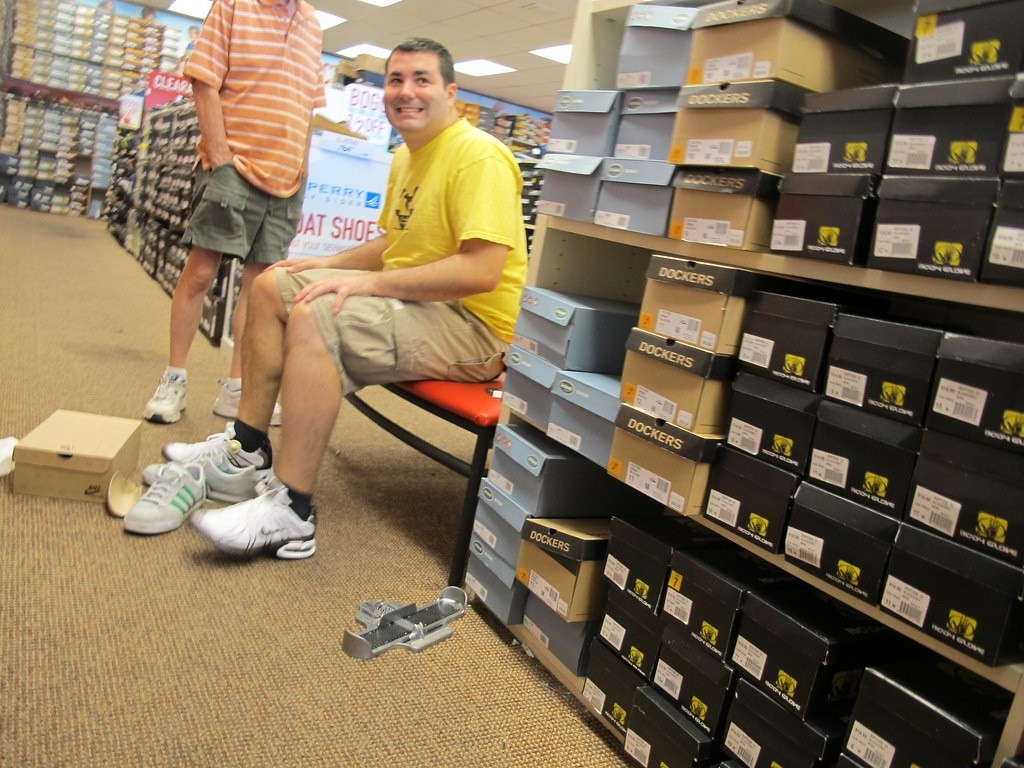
[163, 37, 528, 557]
[142, 0, 328, 426]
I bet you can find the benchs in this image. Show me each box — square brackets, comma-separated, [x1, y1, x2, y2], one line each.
[342, 370, 512, 589]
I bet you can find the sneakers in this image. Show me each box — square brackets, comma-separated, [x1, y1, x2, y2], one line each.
[142, 422, 274, 502]
[213, 378, 281, 427]
[123, 463, 207, 535]
[143, 368, 188, 424]
[191, 473, 318, 559]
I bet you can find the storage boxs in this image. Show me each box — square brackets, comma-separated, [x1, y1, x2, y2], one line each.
[1, 0, 1024, 768]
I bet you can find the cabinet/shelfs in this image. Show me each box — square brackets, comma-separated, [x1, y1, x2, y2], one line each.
[458, 2, 1024, 768]
